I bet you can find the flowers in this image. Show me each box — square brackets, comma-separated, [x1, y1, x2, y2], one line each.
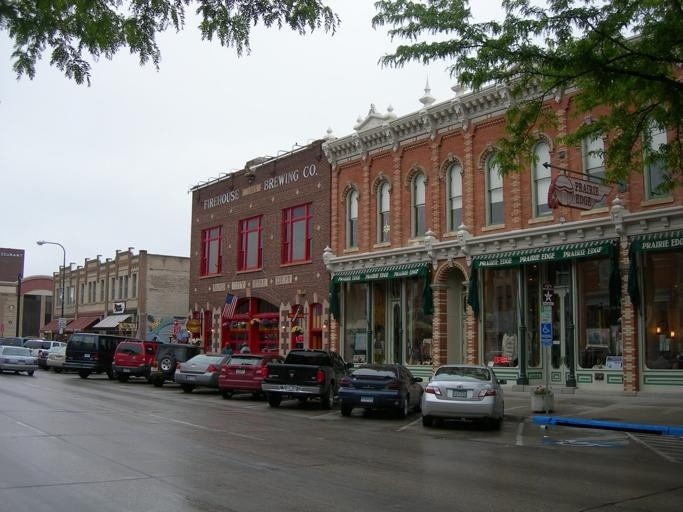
[222, 319, 304, 348]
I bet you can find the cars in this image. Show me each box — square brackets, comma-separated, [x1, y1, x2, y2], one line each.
[171, 349, 230, 397]
[418, 363, 506, 428]
[334, 360, 424, 421]
[217, 351, 284, 402]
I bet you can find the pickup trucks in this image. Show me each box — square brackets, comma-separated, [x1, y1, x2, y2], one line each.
[262, 347, 350, 411]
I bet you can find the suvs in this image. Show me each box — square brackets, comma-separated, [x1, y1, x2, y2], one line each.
[1, 332, 202, 392]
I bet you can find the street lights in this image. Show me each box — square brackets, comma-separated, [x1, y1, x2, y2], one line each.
[35, 239, 67, 321]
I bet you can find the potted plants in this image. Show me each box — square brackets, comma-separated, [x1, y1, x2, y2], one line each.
[530, 385, 555, 411]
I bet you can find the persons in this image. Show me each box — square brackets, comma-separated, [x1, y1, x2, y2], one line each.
[221, 343, 232, 354]
[239, 342, 250, 353]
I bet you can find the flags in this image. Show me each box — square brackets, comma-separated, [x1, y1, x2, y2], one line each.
[220, 292, 238, 319]
